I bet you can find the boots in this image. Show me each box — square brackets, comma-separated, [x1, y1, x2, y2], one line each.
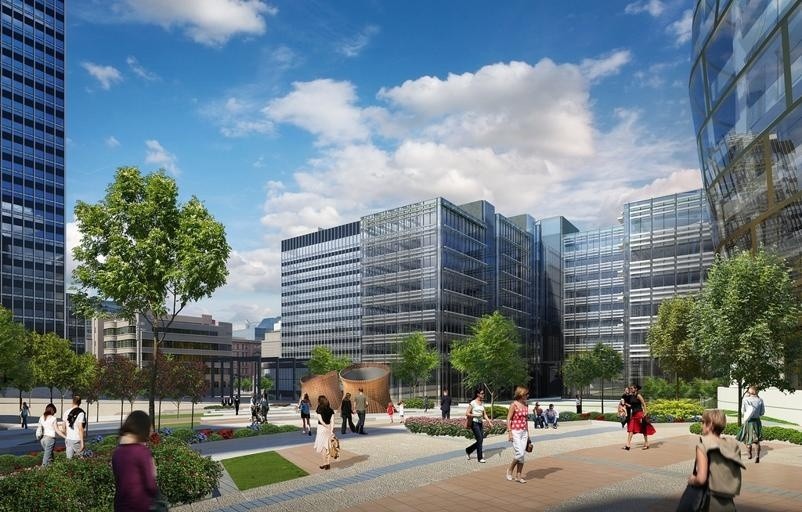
[747, 445, 760, 463]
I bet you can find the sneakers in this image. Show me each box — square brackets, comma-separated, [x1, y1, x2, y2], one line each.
[302, 430, 311, 436]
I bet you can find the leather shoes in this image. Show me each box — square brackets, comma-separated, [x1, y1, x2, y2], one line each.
[466, 447, 486, 463]
[507, 468, 526, 484]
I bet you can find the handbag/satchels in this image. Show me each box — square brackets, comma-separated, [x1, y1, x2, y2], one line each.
[677, 475, 710, 512]
[465, 416, 473, 429]
[527, 440, 533, 453]
[34, 424, 43, 440]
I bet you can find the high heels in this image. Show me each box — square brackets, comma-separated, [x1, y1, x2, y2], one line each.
[320, 464, 328, 469]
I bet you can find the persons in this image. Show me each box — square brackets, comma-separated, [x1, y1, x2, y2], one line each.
[621, 383, 656, 450]
[533, 402, 559, 428]
[112, 410, 160, 512]
[687, 410, 746, 512]
[576, 394, 581, 414]
[19, 402, 31, 429]
[506, 386, 530, 483]
[249, 393, 269, 424]
[228, 393, 240, 416]
[620, 386, 633, 428]
[387, 400, 405, 423]
[356, 387, 368, 434]
[439, 390, 451, 419]
[340, 393, 356, 433]
[465, 385, 492, 463]
[299, 393, 312, 436]
[741, 386, 765, 462]
[38, 404, 66, 465]
[62, 396, 87, 459]
[316, 396, 335, 469]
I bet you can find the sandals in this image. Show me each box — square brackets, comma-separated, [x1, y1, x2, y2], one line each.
[622, 445, 650, 450]
